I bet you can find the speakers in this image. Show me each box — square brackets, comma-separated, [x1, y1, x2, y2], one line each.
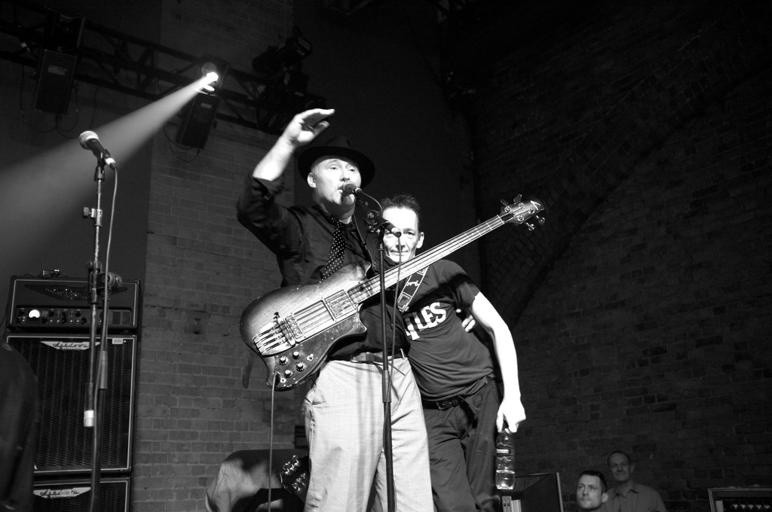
[30, 476, 131, 512]
[6, 334, 136, 478]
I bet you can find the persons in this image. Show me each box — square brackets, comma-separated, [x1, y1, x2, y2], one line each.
[563, 470, 608, 512]
[235, 108, 480, 512]
[592, 449, 667, 511]
[367, 194, 529, 511]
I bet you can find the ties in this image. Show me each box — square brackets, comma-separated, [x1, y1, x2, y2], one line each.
[322, 222, 346, 279]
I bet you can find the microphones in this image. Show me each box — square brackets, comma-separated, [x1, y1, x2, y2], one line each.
[366, 207, 401, 241]
[77, 127, 117, 169]
[342, 182, 382, 212]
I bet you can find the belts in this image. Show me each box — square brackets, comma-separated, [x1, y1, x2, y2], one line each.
[350, 350, 407, 363]
[423, 393, 469, 411]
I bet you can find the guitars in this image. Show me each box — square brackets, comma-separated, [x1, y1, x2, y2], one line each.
[238, 195, 547, 391]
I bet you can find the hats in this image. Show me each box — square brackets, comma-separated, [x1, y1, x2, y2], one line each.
[297, 136, 376, 189]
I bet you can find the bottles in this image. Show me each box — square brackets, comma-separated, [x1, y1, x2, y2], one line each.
[495, 420, 518, 490]
